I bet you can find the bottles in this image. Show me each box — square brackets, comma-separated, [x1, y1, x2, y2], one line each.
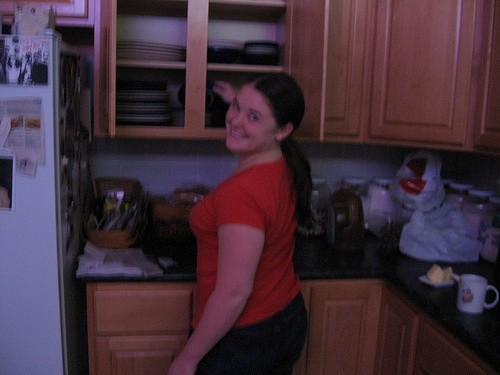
[479, 197, 500, 262]
[341, 177, 368, 196]
[449, 181, 471, 194]
[372, 176, 393, 189]
[463, 190, 492, 225]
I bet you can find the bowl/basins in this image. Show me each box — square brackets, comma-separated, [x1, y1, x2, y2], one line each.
[207, 38, 241, 63]
[242, 39, 281, 65]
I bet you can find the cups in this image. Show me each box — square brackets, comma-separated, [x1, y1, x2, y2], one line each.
[456, 274, 499, 315]
[166, 82, 185, 107]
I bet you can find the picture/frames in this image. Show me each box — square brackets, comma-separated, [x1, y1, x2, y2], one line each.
[0, 0, 88, 17]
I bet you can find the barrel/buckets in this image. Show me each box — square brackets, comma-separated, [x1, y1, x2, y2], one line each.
[82, 175, 149, 249]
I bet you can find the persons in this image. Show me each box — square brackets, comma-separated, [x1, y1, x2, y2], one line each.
[0, 35, 49, 85]
[167, 73, 317, 375]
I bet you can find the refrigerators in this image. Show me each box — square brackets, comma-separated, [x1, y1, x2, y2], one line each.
[0, 29, 92, 375]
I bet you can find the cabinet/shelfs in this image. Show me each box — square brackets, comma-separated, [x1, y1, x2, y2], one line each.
[96, 0, 500, 157]
[86, 278, 494, 375]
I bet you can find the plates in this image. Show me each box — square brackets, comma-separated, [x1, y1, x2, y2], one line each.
[418, 274, 460, 287]
[116, 40, 186, 61]
[116, 80, 170, 126]
